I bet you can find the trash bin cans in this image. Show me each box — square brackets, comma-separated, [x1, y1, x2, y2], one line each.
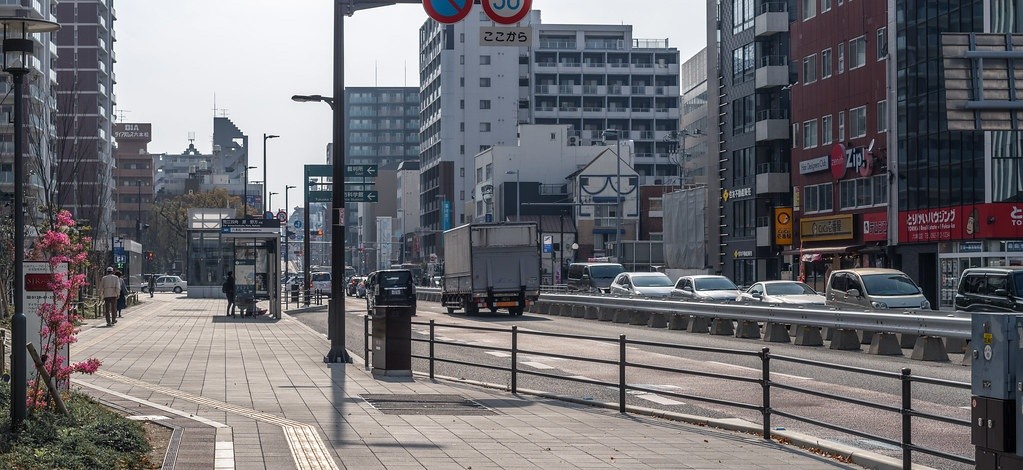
[291, 284, 299, 302]
[372, 306, 411, 370]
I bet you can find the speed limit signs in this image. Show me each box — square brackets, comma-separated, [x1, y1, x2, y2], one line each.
[482, 0, 532, 24]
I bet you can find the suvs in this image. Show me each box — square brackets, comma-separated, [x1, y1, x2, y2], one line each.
[954, 266, 1023, 314]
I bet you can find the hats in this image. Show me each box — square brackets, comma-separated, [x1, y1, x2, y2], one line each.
[107, 266, 114, 272]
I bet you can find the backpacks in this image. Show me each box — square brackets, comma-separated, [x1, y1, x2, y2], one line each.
[222, 282, 230, 292]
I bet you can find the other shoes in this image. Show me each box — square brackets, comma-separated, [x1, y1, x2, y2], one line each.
[112, 320, 118, 324]
[107, 323, 112, 327]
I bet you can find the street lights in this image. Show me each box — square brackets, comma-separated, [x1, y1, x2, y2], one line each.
[263, 134, 280, 219]
[285, 185, 296, 276]
[605, 129, 620, 263]
[505, 170, 520, 221]
[244, 165, 257, 219]
[291, 95, 346, 363]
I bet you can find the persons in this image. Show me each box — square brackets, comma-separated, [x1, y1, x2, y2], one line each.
[226, 271, 235, 316]
[148, 274, 157, 298]
[99, 267, 120, 326]
[114, 271, 128, 317]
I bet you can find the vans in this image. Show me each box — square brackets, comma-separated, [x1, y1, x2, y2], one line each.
[567, 263, 625, 294]
[825, 268, 932, 312]
[366, 269, 417, 315]
[310, 272, 331, 299]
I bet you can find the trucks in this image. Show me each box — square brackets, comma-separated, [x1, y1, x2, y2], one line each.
[440, 221, 541, 315]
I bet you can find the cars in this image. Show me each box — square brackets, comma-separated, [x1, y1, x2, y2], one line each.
[141, 276, 187, 293]
[346, 277, 361, 296]
[355, 278, 367, 298]
[281, 272, 304, 286]
[735, 281, 826, 306]
[670, 275, 741, 301]
[610, 272, 674, 301]
